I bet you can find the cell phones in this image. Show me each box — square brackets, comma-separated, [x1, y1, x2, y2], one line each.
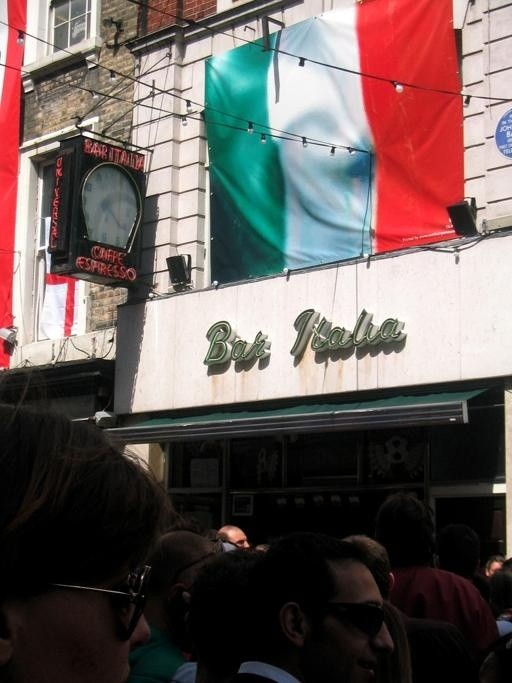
[168, 587, 191, 653]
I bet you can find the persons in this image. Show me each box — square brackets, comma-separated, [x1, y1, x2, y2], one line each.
[124, 494, 510, 681]
[0, 405, 169, 683]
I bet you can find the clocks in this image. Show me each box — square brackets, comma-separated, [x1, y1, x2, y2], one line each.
[81, 160, 144, 256]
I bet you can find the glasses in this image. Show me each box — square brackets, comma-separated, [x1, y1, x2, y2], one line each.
[299, 599, 386, 636]
[44, 562, 153, 641]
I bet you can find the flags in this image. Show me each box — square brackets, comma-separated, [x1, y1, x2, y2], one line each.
[205, 1, 463, 283]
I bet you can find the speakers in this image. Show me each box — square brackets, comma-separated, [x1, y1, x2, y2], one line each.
[447, 202, 478, 237]
[166, 255, 189, 283]
